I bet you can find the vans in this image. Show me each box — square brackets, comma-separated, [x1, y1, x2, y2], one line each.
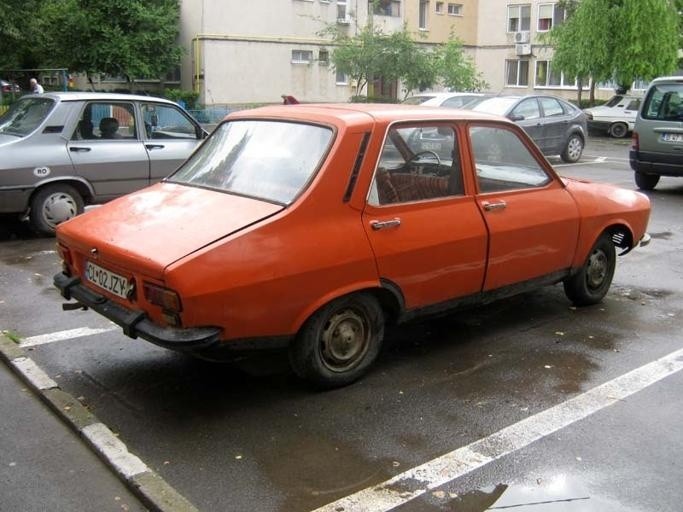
[628, 75, 682, 190]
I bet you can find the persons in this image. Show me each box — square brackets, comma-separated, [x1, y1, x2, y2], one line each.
[29, 78, 45, 94]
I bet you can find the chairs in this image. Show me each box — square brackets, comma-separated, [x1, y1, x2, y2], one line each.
[388, 171, 450, 198]
[98, 117, 122, 139]
[77, 120, 97, 139]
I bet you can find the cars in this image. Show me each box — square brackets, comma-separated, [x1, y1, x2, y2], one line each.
[410, 93, 588, 171]
[0, 89, 215, 239]
[51, 102, 651, 391]
[583, 93, 646, 138]
[380, 93, 493, 155]
[0, 80, 20, 94]
